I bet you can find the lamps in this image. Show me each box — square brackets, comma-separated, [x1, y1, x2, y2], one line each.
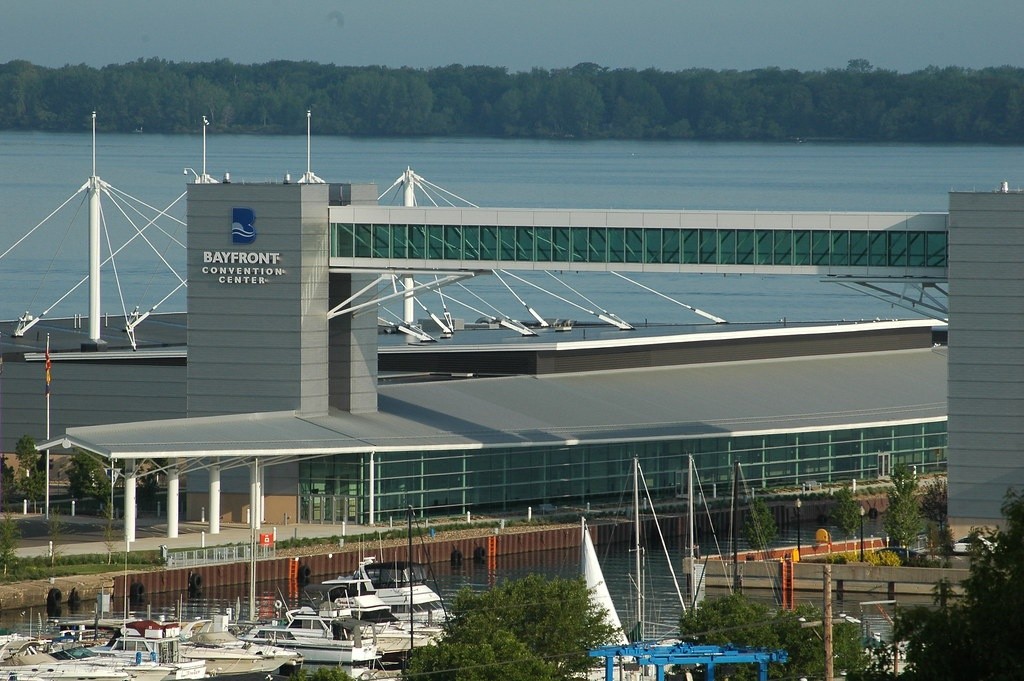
[62, 438, 71, 448]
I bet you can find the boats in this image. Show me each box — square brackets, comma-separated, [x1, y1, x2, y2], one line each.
[0, 554, 454, 681]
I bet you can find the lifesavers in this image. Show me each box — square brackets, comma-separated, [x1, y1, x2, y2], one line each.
[274, 600, 282, 609]
